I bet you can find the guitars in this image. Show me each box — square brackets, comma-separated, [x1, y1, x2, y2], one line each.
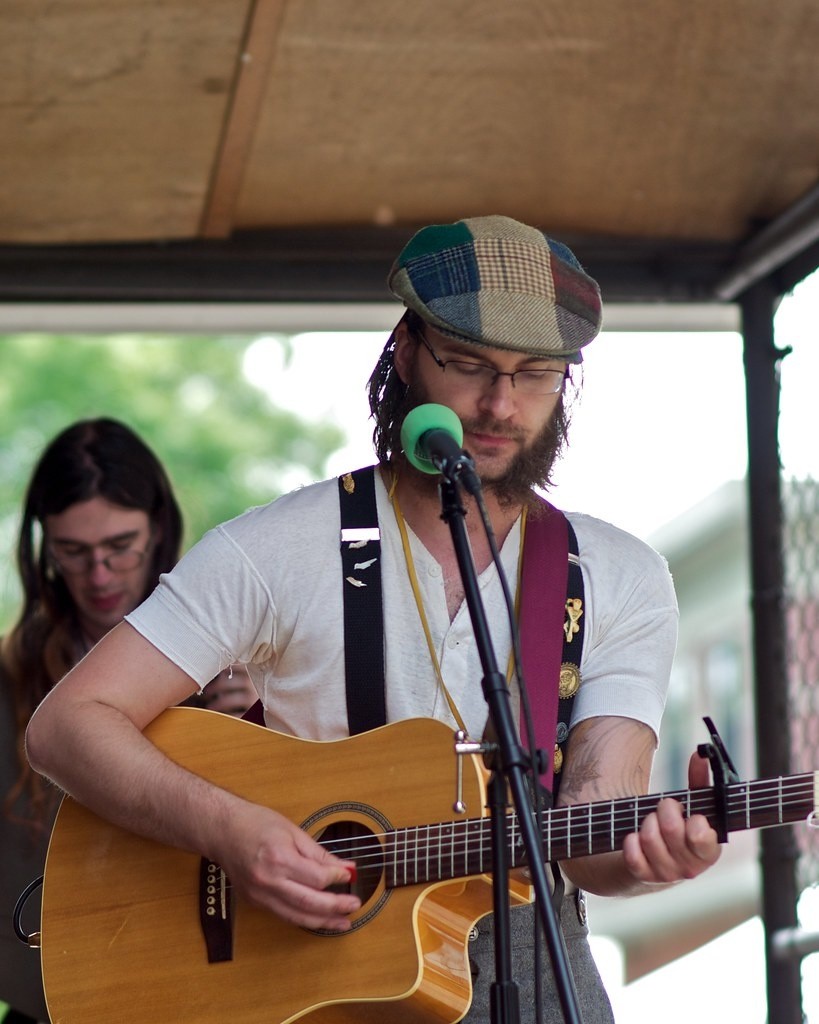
[33, 703, 819, 1024]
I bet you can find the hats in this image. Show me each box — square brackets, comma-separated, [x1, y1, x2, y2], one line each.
[388, 215, 602, 364]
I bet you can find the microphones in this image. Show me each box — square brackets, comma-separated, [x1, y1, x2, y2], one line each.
[401, 403, 479, 499]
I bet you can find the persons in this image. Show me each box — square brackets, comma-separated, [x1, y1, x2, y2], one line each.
[0, 417, 271, 1024]
[24, 214, 722, 1024]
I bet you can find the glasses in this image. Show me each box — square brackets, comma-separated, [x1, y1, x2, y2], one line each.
[45, 529, 153, 576]
[417, 328, 570, 394]
